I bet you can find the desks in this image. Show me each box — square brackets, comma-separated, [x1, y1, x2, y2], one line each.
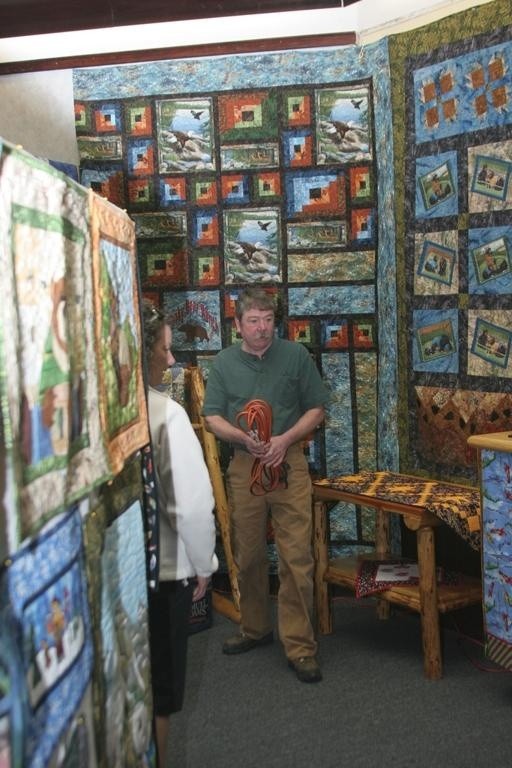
[307, 468, 482, 681]
[464, 427, 511, 677]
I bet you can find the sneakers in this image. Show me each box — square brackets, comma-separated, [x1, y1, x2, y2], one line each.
[223, 630, 273, 654]
[288, 651, 323, 682]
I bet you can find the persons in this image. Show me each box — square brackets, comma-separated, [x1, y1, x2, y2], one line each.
[200, 287, 327, 683]
[424, 164, 500, 355]
[142, 306, 219, 768]
